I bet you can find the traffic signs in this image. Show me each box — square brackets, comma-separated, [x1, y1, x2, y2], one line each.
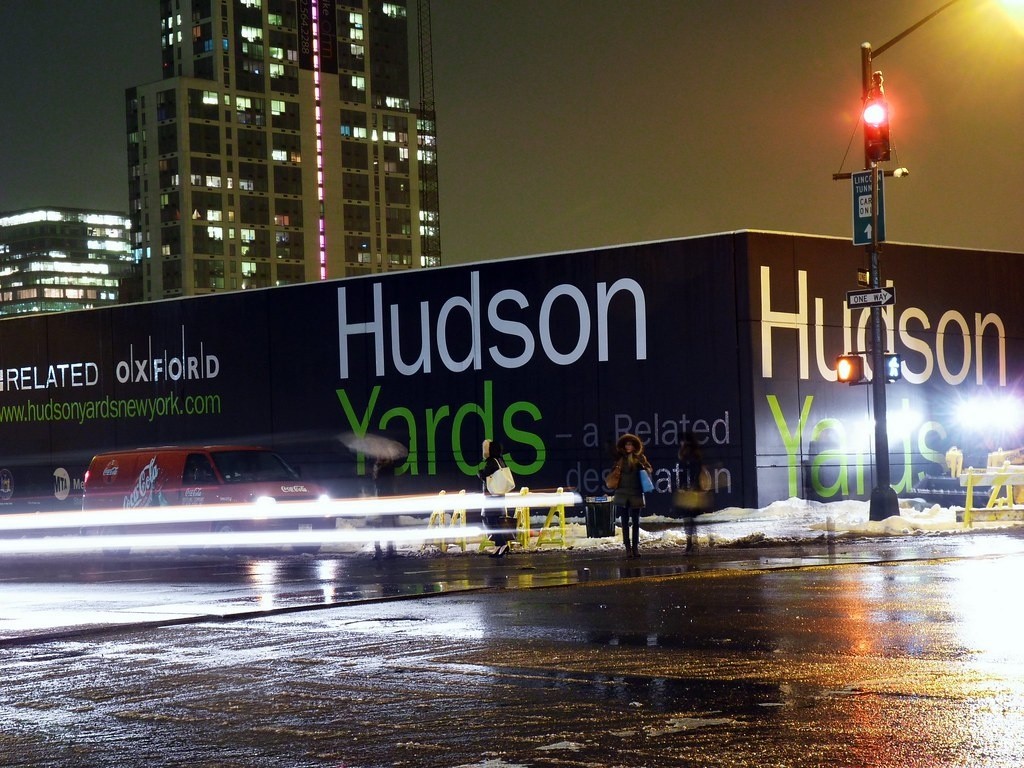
[847, 287, 896, 308]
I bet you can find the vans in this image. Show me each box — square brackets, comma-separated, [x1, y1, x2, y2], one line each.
[81, 445, 338, 554]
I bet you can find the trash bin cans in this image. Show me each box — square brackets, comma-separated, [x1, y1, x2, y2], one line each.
[585, 499, 617, 538]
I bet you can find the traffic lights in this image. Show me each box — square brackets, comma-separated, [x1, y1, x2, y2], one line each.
[837, 358, 863, 385]
[885, 355, 901, 380]
[863, 100, 890, 162]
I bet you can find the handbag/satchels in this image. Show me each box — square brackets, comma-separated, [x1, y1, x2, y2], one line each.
[640, 462, 655, 493]
[486, 458, 515, 497]
[605, 457, 625, 489]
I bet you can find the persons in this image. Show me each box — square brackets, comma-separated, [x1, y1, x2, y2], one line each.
[477, 440, 509, 559]
[613, 433, 653, 557]
[366, 456, 396, 559]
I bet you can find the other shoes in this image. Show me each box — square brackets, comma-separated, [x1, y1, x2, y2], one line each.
[626, 545, 633, 558]
[632, 547, 641, 557]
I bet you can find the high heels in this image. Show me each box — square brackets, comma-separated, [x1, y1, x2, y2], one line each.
[488, 545, 510, 558]
